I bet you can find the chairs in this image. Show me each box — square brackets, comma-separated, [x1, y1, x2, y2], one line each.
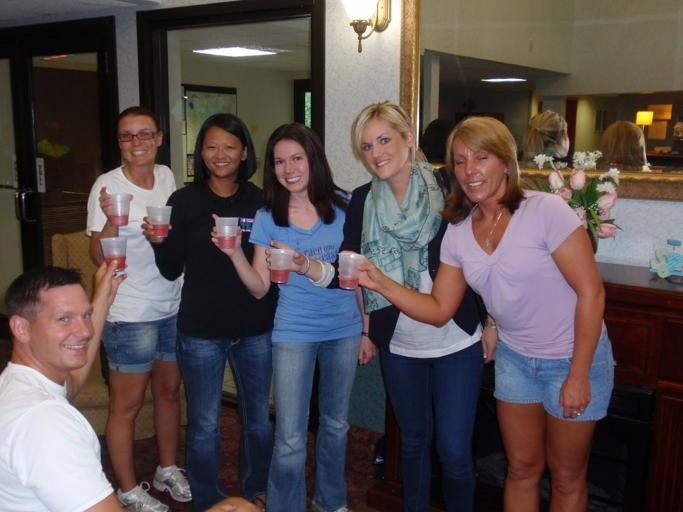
[48, 229, 192, 442]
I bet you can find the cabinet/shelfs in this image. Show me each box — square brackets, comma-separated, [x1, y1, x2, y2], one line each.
[366, 260, 682, 510]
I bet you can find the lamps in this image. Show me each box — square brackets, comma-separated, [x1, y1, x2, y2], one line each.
[634, 111, 653, 130]
[340, 0, 391, 52]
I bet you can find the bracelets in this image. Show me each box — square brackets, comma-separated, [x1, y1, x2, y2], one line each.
[484, 319, 497, 329]
[362, 330, 369, 337]
[297, 253, 310, 275]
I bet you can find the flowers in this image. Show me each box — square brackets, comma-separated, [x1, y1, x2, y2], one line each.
[530, 149, 624, 254]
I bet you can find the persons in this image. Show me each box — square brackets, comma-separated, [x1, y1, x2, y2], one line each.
[598, 119, 650, 172]
[141, 111, 279, 512]
[0, 257, 262, 512]
[210, 122, 377, 511]
[85, 107, 194, 511]
[264, 101, 496, 511]
[419, 119, 454, 164]
[337, 117, 616, 512]
[521, 110, 570, 168]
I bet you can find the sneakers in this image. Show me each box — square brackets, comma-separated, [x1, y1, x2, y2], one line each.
[117, 485, 169, 512]
[153, 465, 192, 503]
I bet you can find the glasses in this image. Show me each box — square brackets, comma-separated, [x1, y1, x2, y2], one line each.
[117, 131, 158, 142]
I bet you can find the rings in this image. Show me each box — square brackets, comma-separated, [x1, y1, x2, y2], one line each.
[577, 413, 581, 416]
[581, 409, 584, 411]
[571, 410, 577, 414]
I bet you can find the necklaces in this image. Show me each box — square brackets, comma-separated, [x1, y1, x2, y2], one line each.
[483, 205, 505, 248]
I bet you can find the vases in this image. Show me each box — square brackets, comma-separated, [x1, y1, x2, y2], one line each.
[585, 225, 599, 258]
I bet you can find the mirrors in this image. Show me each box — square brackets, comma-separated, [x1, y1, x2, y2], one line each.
[399, 0, 682, 202]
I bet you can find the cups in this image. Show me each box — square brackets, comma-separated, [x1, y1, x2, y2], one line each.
[107, 193, 132, 226]
[98, 237, 126, 272]
[336, 252, 363, 290]
[270, 249, 291, 283]
[145, 206, 172, 237]
[214, 217, 239, 248]
